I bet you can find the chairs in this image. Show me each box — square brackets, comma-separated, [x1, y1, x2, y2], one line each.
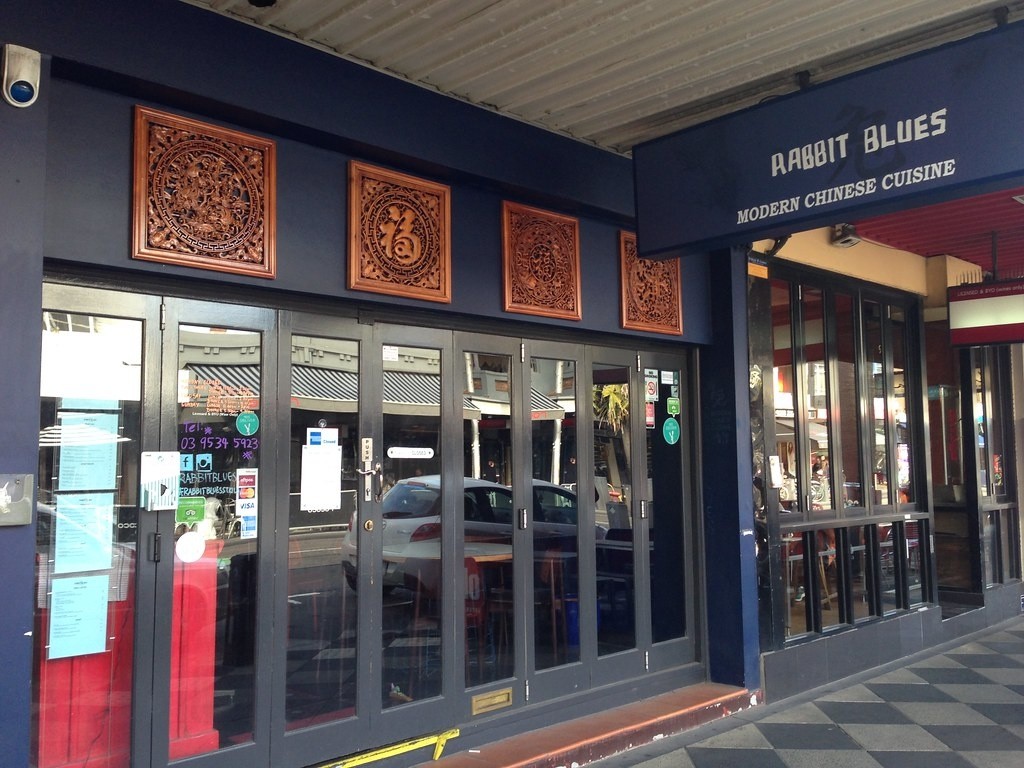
[227, 534, 578, 708]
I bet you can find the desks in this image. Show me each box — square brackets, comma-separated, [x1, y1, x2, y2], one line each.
[380, 543, 515, 682]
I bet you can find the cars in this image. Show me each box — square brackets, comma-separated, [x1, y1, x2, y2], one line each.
[35, 501, 231, 648]
[342, 472, 608, 622]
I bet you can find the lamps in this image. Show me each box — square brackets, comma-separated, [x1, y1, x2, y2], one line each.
[0, 43, 42, 109]
[830, 223, 861, 249]
